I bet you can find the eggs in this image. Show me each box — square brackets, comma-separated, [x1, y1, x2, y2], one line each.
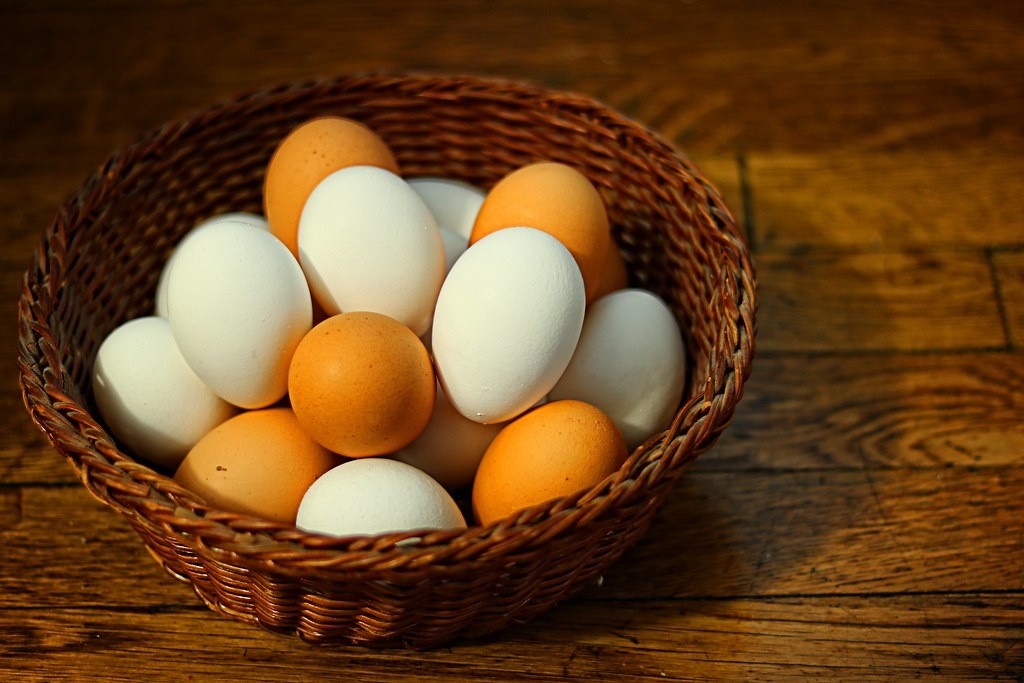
[89, 117, 688, 536]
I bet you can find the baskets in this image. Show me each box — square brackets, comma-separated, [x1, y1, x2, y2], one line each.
[13, 65, 760, 646]
